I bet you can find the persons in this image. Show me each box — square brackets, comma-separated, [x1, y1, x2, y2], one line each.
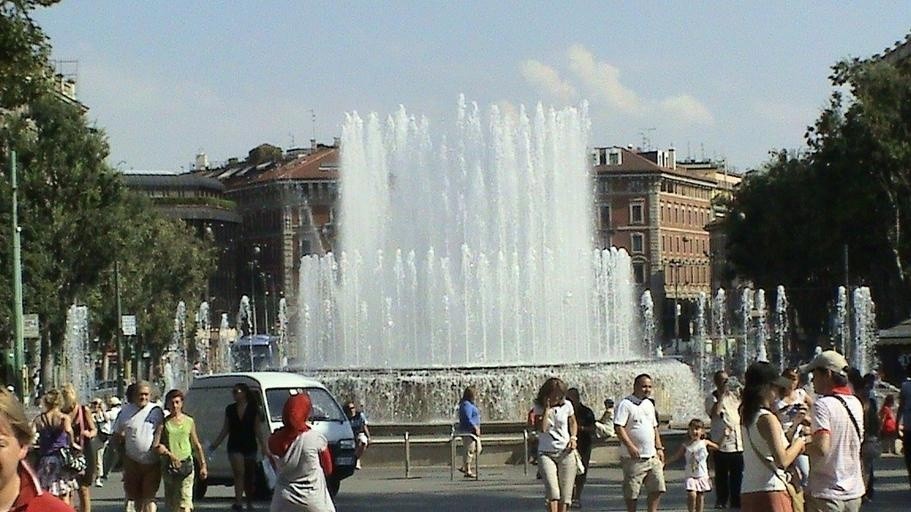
[895, 363, 911, 492]
[703, 370, 745, 509]
[29, 385, 83, 505]
[594, 398, 616, 441]
[531, 377, 579, 511]
[875, 392, 905, 439]
[773, 365, 813, 487]
[737, 360, 809, 512]
[565, 388, 595, 508]
[458, 385, 482, 478]
[207, 382, 267, 512]
[342, 401, 372, 470]
[150, 388, 209, 512]
[846, 366, 865, 396]
[0, 384, 78, 512]
[612, 373, 666, 511]
[103, 379, 165, 512]
[862, 372, 876, 390]
[857, 383, 878, 505]
[661, 418, 720, 512]
[101, 395, 123, 479]
[87, 396, 110, 488]
[798, 350, 867, 512]
[263, 392, 336, 512]
[6, 383, 19, 403]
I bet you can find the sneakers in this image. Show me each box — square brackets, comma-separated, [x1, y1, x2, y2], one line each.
[356, 459, 361, 469]
[95, 478, 103, 487]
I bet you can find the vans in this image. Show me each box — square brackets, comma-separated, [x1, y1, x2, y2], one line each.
[167, 372, 361, 498]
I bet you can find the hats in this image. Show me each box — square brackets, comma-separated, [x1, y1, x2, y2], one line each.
[799, 350, 848, 377]
[107, 397, 122, 406]
[745, 361, 783, 387]
[89, 397, 102, 404]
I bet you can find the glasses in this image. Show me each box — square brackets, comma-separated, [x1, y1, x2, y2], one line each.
[232, 389, 243, 393]
[171, 401, 182, 404]
[349, 407, 355, 410]
[137, 391, 150, 397]
[637, 380, 652, 388]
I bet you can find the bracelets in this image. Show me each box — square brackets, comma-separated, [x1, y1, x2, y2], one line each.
[656, 446, 665, 451]
[199, 461, 207, 468]
[570, 436, 578, 440]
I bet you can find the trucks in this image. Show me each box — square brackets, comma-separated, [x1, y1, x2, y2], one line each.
[223, 338, 283, 374]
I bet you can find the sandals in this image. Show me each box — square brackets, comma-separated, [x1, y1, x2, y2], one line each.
[458, 467, 466, 472]
[464, 472, 476, 478]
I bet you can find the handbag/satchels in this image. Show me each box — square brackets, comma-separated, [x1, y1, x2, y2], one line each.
[59, 446, 88, 471]
[261, 456, 277, 491]
[97, 421, 111, 441]
[84, 437, 97, 473]
[169, 456, 191, 482]
[786, 469, 806, 512]
[861, 436, 883, 457]
[574, 449, 585, 476]
